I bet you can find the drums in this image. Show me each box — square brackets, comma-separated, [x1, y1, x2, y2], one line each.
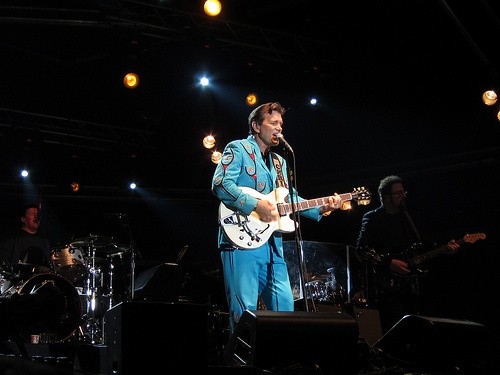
[2, 272, 82, 344]
[0, 270, 21, 296]
[48, 244, 88, 282]
[305, 281, 329, 303]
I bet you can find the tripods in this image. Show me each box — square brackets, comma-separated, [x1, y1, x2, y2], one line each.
[64, 245, 103, 345]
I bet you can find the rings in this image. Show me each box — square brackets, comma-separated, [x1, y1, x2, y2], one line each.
[335, 204, 337, 206]
[400, 269, 401, 272]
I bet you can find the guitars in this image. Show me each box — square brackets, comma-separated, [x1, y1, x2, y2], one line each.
[217, 186, 371, 248]
[370, 232, 487, 290]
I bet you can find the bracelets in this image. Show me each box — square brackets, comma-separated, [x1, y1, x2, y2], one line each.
[253, 202, 258, 211]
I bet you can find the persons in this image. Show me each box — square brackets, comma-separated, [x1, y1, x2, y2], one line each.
[212, 103, 343, 336]
[357, 175, 460, 337]
[0, 204, 51, 269]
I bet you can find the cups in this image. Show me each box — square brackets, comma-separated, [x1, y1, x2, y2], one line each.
[31, 335, 39, 344]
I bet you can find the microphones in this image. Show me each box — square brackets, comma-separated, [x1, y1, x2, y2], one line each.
[276, 133, 293, 153]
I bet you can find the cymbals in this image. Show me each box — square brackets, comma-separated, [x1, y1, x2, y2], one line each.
[71, 235, 113, 246]
[91, 242, 130, 257]
[5, 262, 31, 265]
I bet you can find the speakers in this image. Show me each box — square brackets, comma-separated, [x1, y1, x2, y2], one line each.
[294, 300, 382, 354]
[223, 311, 359, 370]
[369, 314, 489, 372]
[104, 302, 210, 375]
